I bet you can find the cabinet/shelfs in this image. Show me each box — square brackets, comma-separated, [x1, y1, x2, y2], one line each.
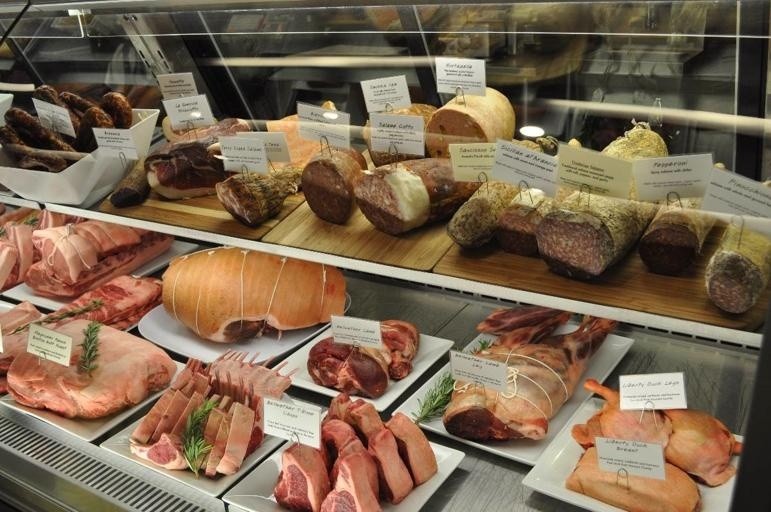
[0, 0, 771, 512]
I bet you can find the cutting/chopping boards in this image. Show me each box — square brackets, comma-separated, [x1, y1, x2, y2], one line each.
[433, 231, 770, 337]
[261, 200, 454, 272]
[97, 189, 305, 242]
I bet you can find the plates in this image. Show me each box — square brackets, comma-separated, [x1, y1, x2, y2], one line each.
[137, 291, 353, 367]
[1, 359, 185, 442]
[269, 315, 456, 413]
[1, 300, 51, 393]
[221, 409, 465, 511]
[99, 399, 323, 499]
[0, 239, 200, 313]
[115, 273, 166, 333]
[520, 398, 744, 512]
[390, 306, 636, 466]
